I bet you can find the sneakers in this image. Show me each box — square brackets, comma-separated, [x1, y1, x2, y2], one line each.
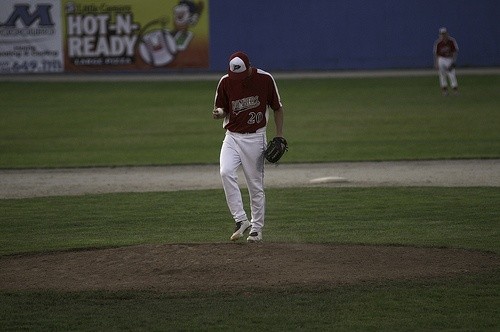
[230, 219, 253, 241]
[453, 92, 460, 97]
[444, 91, 449, 96]
[247, 231, 263, 243]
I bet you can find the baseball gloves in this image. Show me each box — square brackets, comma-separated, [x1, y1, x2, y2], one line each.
[262, 136, 289, 164]
[447, 60, 458, 73]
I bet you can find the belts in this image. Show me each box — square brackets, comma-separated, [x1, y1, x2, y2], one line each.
[239, 130, 257, 134]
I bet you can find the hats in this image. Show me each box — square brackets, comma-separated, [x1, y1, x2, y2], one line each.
[439, 28, 448, 35]
[228, 52, 250, 81]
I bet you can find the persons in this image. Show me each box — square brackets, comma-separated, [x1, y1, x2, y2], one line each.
[212, 52, 287, 241]
[434, 28, 460, 96]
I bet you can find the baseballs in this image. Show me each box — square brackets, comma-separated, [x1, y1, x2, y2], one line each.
[214, 107, 224, 119]
